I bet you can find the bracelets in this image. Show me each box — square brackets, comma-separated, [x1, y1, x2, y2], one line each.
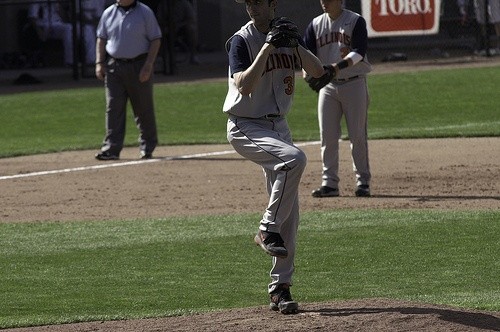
[96, 62, 103, 64]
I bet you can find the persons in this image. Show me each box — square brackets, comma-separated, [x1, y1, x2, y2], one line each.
[458, 0, 500, 55]
[95, 0, 162, 160]
[1, 1, 201, 84]
[303, 0, 371, 197]
[223, 0, 323, 313]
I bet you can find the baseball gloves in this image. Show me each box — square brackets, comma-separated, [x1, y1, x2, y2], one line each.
[308, 63, 337, 94]
[264, 15, 300, 49]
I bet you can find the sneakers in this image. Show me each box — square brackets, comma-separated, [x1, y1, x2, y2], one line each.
[253, 229, 288, 258]
[312, 186, 339, 196]
[269, 288, 298, 312]
[95, 151, 119, 160]
[140, 150, 152, 159]
[355, 185, 370, 196]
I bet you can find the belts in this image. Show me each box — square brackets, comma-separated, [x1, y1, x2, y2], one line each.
[337, 75, 358, 80]
[265, 114, 281, 120]
[114, 58, 132, 64]
